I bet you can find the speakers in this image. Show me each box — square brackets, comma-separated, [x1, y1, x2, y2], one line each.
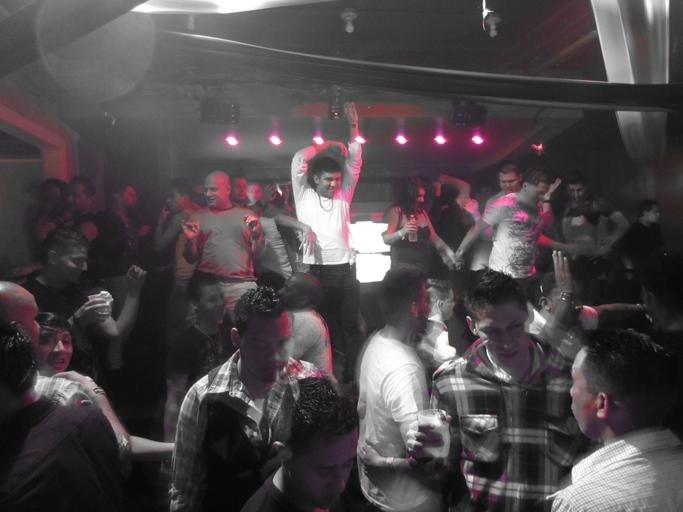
[450, 103, 488, 127]
[201, 103, 240, 125]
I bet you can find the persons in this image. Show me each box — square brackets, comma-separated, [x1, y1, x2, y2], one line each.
[1, 170, 357, 510]
[290, 101, 363, 383]
[357, 162, 682, 510]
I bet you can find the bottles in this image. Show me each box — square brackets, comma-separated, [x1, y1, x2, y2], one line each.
[408, 215, 418, 242]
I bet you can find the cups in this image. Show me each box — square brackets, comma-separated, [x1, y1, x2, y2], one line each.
[87, 292, 112, 317]
[418, 408, 451, 460]
